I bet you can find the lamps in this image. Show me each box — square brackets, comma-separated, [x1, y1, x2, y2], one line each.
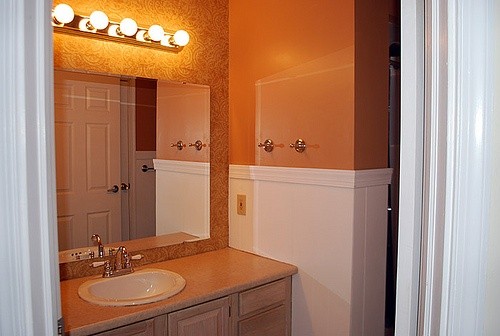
[86, 10, 109, 30]
[168, 28, 190, 49]
[53, 3, 75, 25]
[116, 17, 138, 36]
[142, 24, 165, 43]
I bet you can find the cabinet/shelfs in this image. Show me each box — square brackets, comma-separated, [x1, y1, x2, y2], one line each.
[58, 244, 298, 336]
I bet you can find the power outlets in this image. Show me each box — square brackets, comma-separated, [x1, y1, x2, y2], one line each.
[237, 194, 245, 215]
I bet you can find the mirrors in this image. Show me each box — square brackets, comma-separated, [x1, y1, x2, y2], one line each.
[51, 67, 212, 266]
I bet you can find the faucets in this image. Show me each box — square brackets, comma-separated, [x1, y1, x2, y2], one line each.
[115, 245, 129, 269]
[91, 234, 105, 256]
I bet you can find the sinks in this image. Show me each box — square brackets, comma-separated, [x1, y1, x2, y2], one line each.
[77, 267, 186, 306]
[59, 247, 121, 263]
